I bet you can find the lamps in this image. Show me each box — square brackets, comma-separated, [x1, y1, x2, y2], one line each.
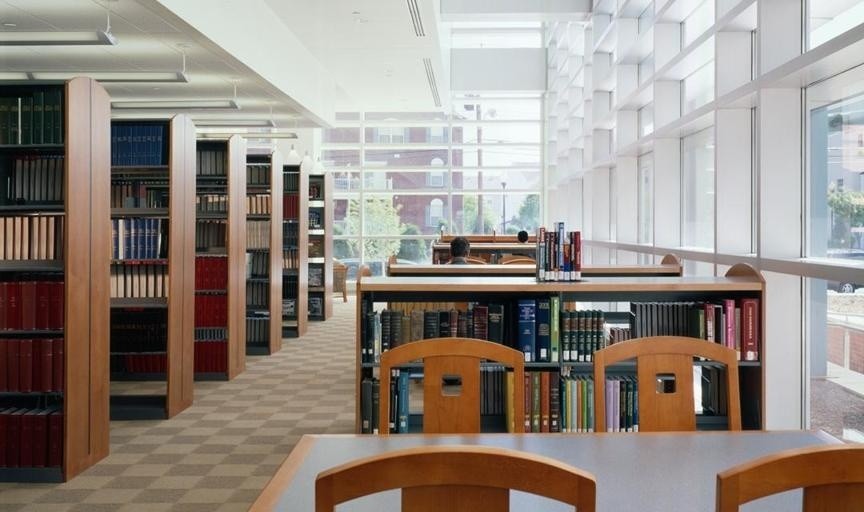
[2, 74, 303, 143]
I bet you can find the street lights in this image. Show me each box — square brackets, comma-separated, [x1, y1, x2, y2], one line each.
[501, 182, 507, 234]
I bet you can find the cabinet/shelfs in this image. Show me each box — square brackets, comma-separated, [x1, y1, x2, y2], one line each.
[429, 233, 571, 265]
[110, 111, 197, 416]
[308, 170, 333, 324]
[196, 132, 247, 382]
[1, 74, 114, 485]
[355, 251, 766, 434]
[283, 158, 310, 340]
[246, 148, 285, 357]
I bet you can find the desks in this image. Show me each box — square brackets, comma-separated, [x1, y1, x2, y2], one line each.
[248, 426, 847, 511]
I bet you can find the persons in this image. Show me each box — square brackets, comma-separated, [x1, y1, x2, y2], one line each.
[451, 237, 471, 264]
[518, 231, 528, 243]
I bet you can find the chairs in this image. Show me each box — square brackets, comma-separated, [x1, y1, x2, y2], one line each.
[333, 266, 349, 303]
[716, 442, 864, 511]
[593, 335, 744, 434]
[377, 336, 526, 433]
[316, 443, 599, 511]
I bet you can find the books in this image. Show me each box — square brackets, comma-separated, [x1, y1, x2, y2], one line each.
[283, 172, 299, 320]
[194, 141, 229, 374]
[0, 89, 65, 467]
[110, 120, 170, 376]
[308, 181, 322, 316]
[536, 222, 582, 282]
[245, 164, 272, 345]
[362, 297, 759, 431]
